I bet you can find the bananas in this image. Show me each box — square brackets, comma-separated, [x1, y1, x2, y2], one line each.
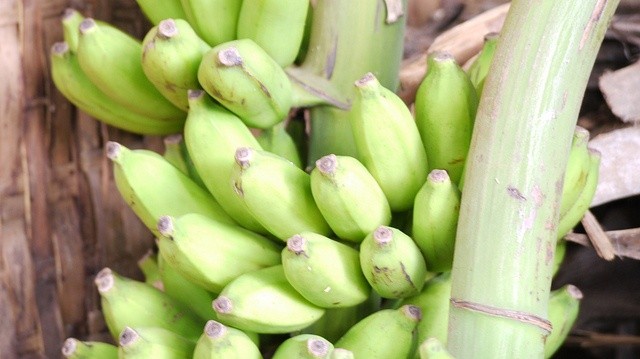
[52, 0, 602, 359]
